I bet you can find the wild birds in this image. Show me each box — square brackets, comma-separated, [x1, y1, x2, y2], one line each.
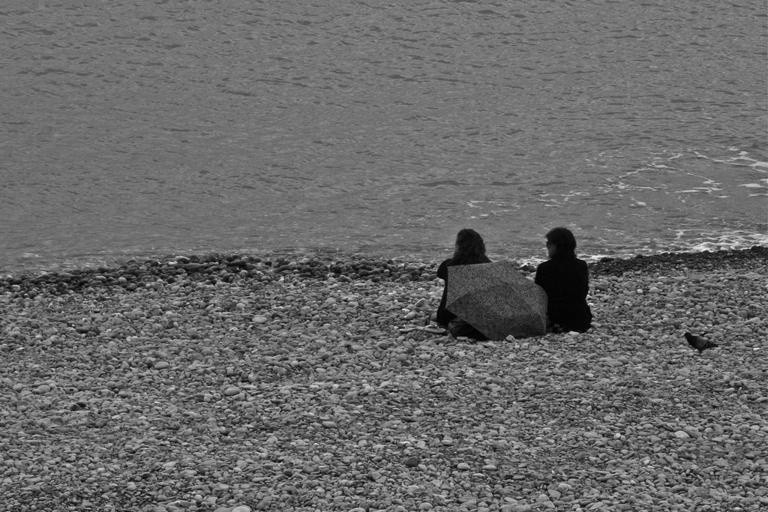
[684, 333, 719, 356]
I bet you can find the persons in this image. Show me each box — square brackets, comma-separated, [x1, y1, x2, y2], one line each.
[535, 228, 592, 332]
[437, 230, 491, 335]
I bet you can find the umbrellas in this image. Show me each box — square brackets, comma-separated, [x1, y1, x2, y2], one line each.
[445, 260, 547, 340]
